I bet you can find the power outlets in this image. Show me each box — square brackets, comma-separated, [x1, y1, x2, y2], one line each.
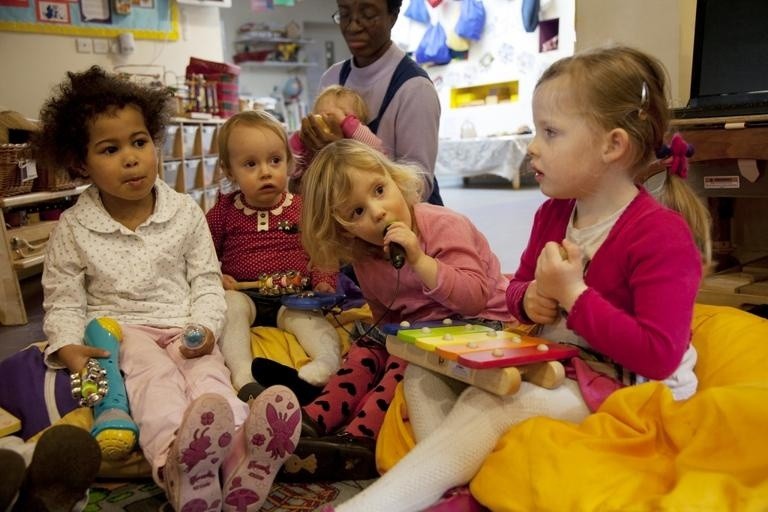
[76, 37, 92, 53]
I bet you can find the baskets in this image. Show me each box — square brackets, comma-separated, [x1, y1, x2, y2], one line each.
[0, 141, 77, 198]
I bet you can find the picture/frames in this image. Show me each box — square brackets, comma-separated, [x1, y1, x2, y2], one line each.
[4, 2, 181, 40]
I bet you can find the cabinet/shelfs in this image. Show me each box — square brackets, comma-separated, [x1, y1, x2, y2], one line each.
[151, 120, 253, 217]
[655, 115, 767, 293]
[0, 118, 93, 326]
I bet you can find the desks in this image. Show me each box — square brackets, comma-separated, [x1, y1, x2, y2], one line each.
[435, 135, 539, 188]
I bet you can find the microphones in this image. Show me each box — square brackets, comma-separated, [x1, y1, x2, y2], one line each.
[385, 225, 405, 269]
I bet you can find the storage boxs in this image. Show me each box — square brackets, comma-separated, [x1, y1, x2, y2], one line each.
[184, 56, 239, 120]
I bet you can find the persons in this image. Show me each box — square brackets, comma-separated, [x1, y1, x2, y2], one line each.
[295, 138, 511, 461]
[320, 0, 443, 200]
[331, 47, 708, 512]
[206, 111, 345, 399]
[39, 65, 303, 512]
[289, 85, 386, 171]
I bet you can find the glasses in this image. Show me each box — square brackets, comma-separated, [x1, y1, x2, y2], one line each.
[331, 10, 389, 28]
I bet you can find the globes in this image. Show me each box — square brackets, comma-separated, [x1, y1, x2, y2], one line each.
[284, 77, 302, 103]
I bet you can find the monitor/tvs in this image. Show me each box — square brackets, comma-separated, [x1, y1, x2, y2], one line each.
[688, 0, 768, 108]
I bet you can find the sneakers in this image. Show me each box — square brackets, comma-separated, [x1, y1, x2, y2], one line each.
[426, 486, 483, 511]
[163, 357, 381, 512]
[1, 424, 105, 510]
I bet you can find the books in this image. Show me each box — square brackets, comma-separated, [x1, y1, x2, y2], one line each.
[702, 256, 768, 296]
